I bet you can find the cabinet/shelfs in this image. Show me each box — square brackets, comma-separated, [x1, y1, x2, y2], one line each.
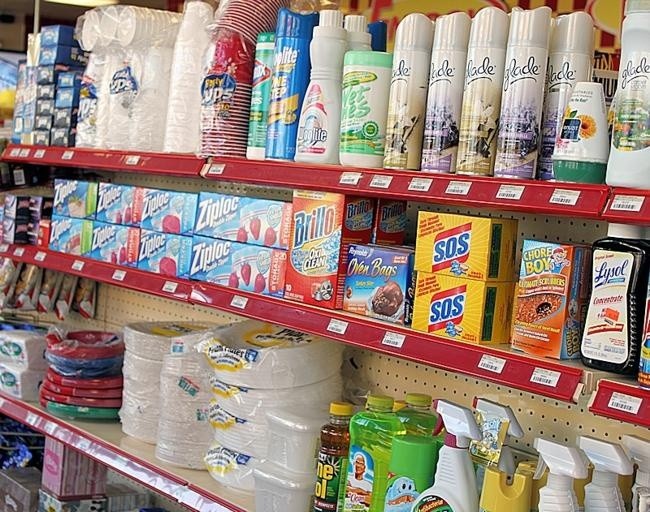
[0, 140, 650, 512]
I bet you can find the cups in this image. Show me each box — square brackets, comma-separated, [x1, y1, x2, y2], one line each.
[77, 0, 319, 161]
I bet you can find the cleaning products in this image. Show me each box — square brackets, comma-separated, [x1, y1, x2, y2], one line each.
[406, 397, 650, 512]
[245, 1, 650, 193]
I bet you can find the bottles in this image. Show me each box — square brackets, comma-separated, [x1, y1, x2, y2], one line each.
[313, 392, 443, 512]
[265, 0, 650, 190]
